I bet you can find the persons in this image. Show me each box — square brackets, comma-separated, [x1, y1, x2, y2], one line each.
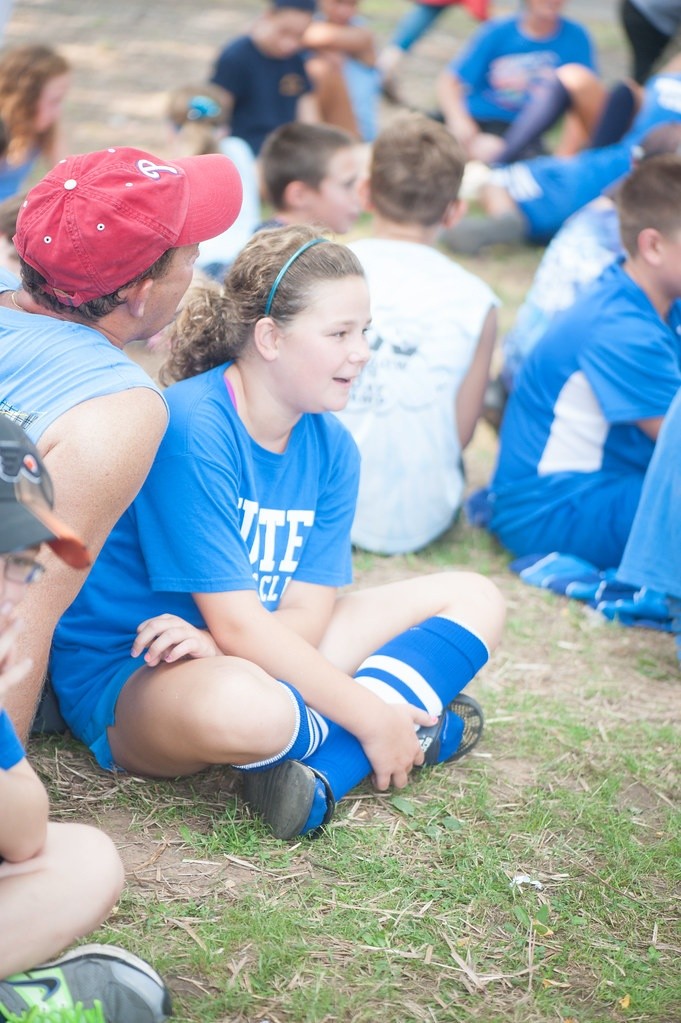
[0, 42, 71, 200]
[147, 0, 681, 636]
[46, 221, 511, 844]
[0, 146, 242, 751]
[0, 414, 171, 1023]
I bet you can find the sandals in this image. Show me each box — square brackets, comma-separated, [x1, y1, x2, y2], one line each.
[410, 695, 484, 765]
[244, 760, 334, 840]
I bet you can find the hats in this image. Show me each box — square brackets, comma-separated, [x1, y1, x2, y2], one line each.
[12, 147, 242, 306]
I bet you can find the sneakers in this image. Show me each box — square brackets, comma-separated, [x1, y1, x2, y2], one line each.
[0, 943, 173, 1023]
